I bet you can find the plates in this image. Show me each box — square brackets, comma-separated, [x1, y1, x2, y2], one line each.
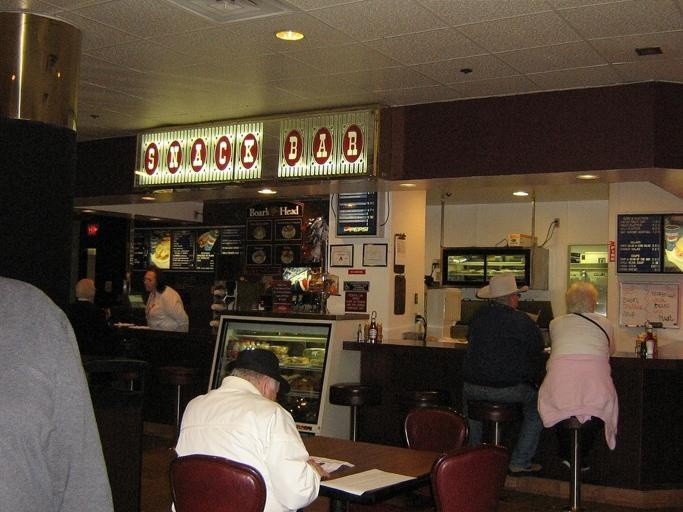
[250, 226, 296, 263]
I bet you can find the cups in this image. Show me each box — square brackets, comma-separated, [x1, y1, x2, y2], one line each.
[204, 232, 218, 252]
[664, 224, 678, 252]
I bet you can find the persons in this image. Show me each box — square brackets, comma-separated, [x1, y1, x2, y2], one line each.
[143, 266, 190, 333]
[458, 272, 548, 477]
[171, 349, 330, 512]
[538, 281, 619, 472]
[64, 279, 115, 359]
[0, 274, 115, 512]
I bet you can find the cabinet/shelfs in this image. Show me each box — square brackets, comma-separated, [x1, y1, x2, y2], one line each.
[216, 321, 330, 431]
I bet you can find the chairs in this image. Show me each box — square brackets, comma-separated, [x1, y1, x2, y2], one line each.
[431, 444, 510, 512]
[404, 408, 468, 454]
[169, 454, 266, 512]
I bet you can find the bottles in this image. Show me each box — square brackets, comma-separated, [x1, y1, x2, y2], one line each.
[289, 283, 320, 314]
[226, 340, 268, 362]
[356, 310, 382, 341]
[635, 323, 658, 357]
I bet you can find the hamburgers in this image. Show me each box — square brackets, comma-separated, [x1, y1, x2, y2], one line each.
[674, 238, 683, 259]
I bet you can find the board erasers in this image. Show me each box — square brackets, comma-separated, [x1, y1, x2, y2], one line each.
[649, 322, 663, 328]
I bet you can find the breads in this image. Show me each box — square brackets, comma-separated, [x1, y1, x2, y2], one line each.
[155, 244, 169, 260]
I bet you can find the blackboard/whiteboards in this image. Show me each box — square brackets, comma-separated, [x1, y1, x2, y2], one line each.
[619, 282, 680, 329]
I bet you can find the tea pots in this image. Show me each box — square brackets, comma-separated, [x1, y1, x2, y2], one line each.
[413, 313, 427, 341]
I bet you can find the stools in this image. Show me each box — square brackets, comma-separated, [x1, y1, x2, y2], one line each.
[330, 381, 382, 442]
[159, 365, 192, 426]
[400, 387, 444, 408]
[554, 417, 591, 511]
[117, 367, 138, 392]
[468, 401, 518, 446]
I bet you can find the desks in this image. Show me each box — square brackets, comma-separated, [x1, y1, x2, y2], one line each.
[303, 435, 439, 512]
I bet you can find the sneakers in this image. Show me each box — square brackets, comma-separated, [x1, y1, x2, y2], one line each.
[508, 464, 543, 476]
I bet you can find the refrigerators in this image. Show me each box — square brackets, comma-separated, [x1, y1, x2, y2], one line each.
[425, 287, 462, 340]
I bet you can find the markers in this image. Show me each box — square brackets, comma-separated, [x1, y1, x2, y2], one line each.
[625, 325, 639, 327]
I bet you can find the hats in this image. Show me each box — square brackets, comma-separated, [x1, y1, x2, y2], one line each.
[224, 349, 290, 394]
[476, 275, 528, 298]
